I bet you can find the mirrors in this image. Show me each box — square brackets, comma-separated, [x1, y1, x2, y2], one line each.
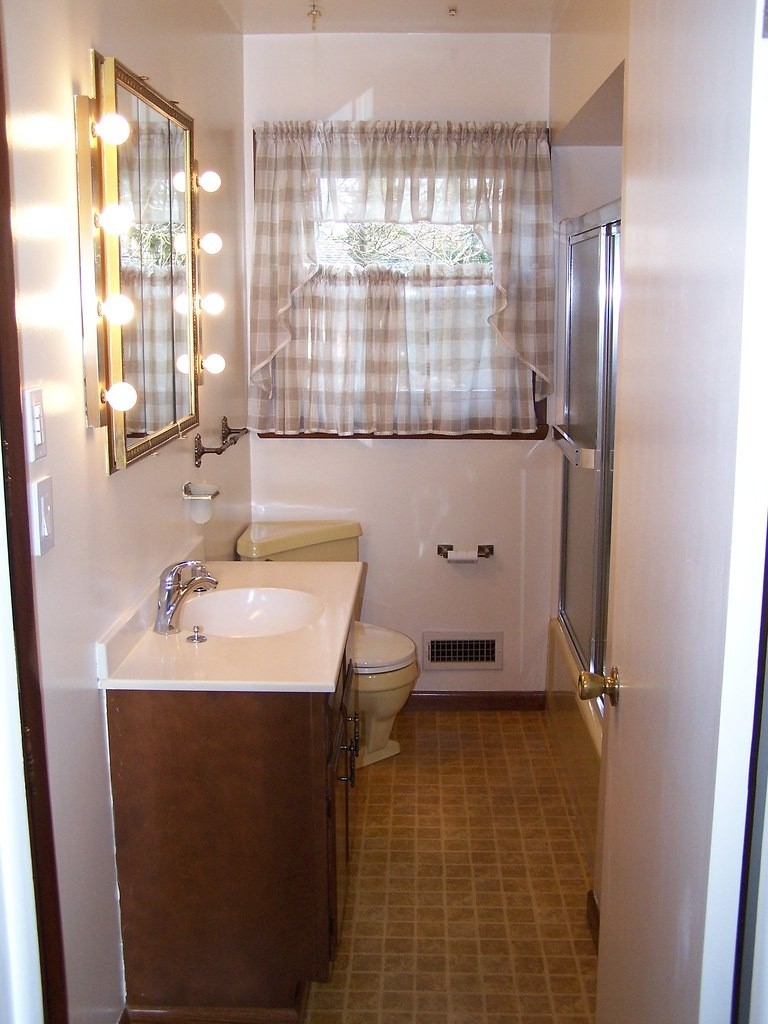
[90, 45, 198, 475]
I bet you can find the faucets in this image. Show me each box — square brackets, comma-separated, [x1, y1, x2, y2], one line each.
[153, 560, 218, 635]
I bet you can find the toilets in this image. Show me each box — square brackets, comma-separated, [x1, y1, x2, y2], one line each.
[237, 520, 421, 770]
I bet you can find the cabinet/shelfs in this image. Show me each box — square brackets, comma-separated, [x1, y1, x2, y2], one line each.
[106, 617, 357, 1023]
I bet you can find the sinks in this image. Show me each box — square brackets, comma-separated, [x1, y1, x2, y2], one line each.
[181, 587, 325, 638]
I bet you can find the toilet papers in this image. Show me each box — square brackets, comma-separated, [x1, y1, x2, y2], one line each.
[447, 551, 479, 561]
